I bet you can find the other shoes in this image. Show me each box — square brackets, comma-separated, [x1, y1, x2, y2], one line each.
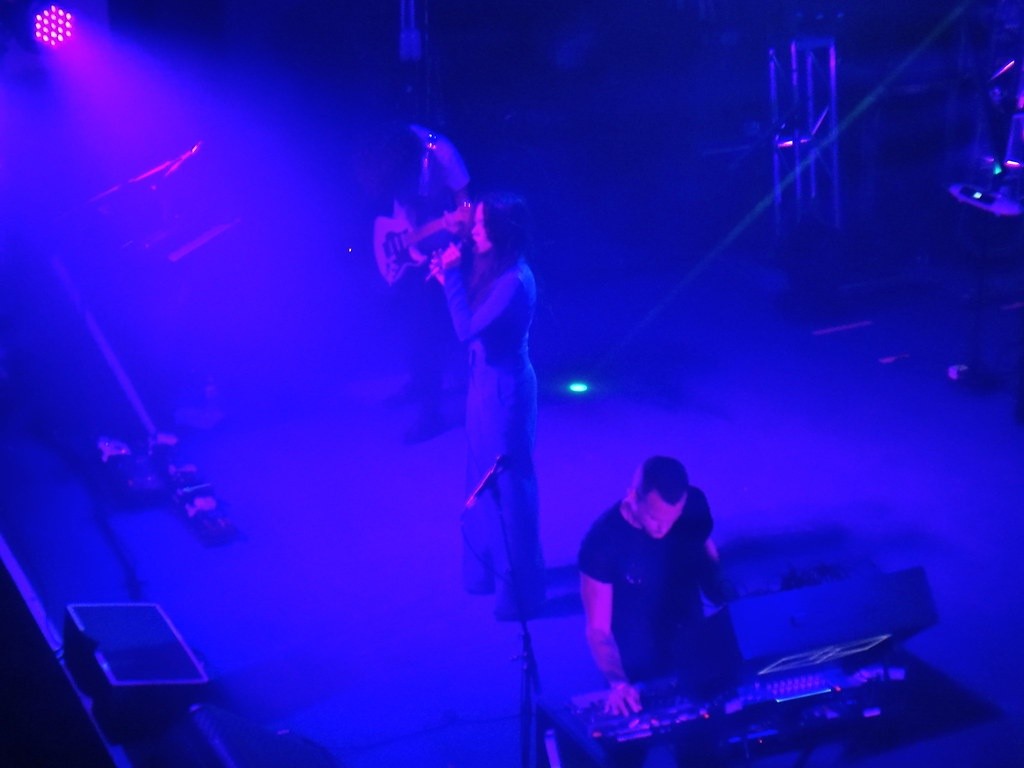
[404, 408, 456, 445]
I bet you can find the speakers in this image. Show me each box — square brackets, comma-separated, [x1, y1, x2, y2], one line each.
[62, 602, 209, 730]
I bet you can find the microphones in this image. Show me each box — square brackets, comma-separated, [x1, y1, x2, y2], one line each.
[467, 453, 511, 509]
[165, 141, 208, 178]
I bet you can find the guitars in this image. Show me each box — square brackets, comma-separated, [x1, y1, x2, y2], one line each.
[372, 201, 474, 286]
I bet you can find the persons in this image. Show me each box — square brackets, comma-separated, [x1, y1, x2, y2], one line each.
[369, 123, 550, 620]
[580, 453, 741, 768]
